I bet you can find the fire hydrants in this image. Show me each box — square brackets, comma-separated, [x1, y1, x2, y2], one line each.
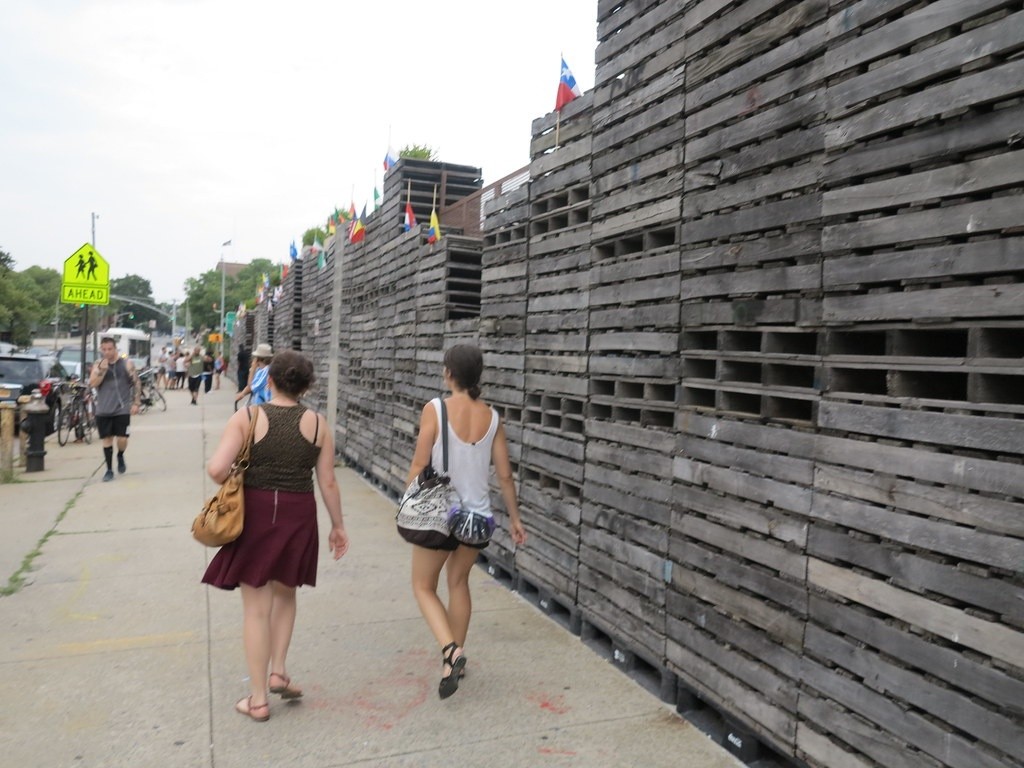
[19, 394, 50, 473]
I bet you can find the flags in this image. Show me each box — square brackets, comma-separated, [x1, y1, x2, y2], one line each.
[255, 265, 289, 312]
[330, 201, 366, 243]
[290, 244, 297, 259]
[383, 145, 397, 171]
[404, 203, 417, 231]
[554, 59, 583, 111]
[236, 304, 246, 317]
[318, 252, 326, 270]
[374, 187, 383, 211]
[428, 211, 441, 245]
[312, 242, 323, 253]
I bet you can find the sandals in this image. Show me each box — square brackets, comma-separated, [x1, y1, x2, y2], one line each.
[234, 695, 271, 722]
[268, 672, 304, 699]
[438, 641, 466, 700]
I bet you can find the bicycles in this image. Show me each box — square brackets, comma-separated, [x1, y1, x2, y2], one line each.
[50, 367, 167, 446]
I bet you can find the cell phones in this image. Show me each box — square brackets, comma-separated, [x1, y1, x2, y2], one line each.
[256, 357, 264, 361]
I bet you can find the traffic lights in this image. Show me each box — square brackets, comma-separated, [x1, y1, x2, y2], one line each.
[128, 303, 138, 320]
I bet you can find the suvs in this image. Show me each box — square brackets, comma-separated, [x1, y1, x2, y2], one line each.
[0, 343, 101, 434]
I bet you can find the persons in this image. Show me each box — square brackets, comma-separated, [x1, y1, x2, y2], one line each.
[209, 353, 348, 724]
[408, 345, 528, 700]
[89, 337, 142, 482]
[158, 347, 225, 405]
[236, 343, 271, 405]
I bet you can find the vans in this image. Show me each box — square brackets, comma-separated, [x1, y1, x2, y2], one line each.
[92, 328, 151, 373]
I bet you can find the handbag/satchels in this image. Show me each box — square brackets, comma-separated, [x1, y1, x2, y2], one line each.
[189, 456, 251, 547]
[395, 467, 461, 547]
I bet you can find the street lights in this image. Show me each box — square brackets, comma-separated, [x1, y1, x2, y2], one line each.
[213, 240, 232, 355]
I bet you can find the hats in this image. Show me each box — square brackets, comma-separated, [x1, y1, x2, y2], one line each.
[250, 343, 275, 357]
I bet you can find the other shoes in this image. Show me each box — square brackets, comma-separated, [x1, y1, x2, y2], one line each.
[102, 470, 113, 482]
[116, 453, 126, 473]
